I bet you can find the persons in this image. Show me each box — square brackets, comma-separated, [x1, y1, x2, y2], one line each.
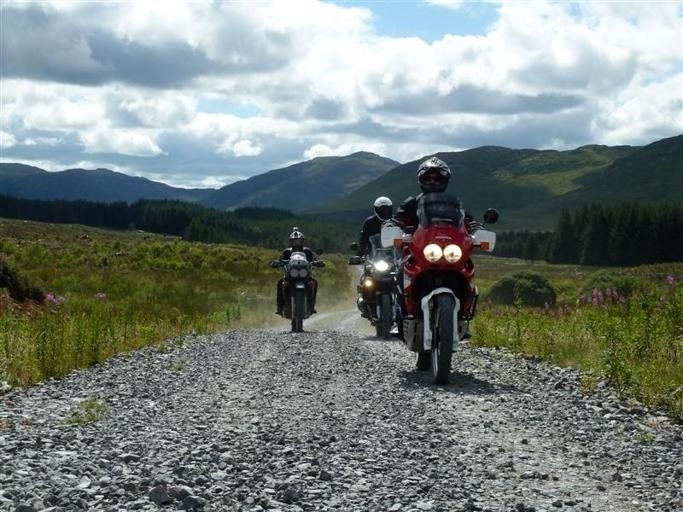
[272, 230, 324, 315]
[392, 157, 485, 340]
[355, 196, 395, 318]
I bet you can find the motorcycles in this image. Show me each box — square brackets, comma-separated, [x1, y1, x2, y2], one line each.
[384, 207, 500, 386]
[272, 250, 326, 332]
[345, 229, 404, 341]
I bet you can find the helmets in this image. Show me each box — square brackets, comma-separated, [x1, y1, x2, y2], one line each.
[416, 157, 451, 193]
[289, 231, 304, 246]
[374, 197, 393, 221]
